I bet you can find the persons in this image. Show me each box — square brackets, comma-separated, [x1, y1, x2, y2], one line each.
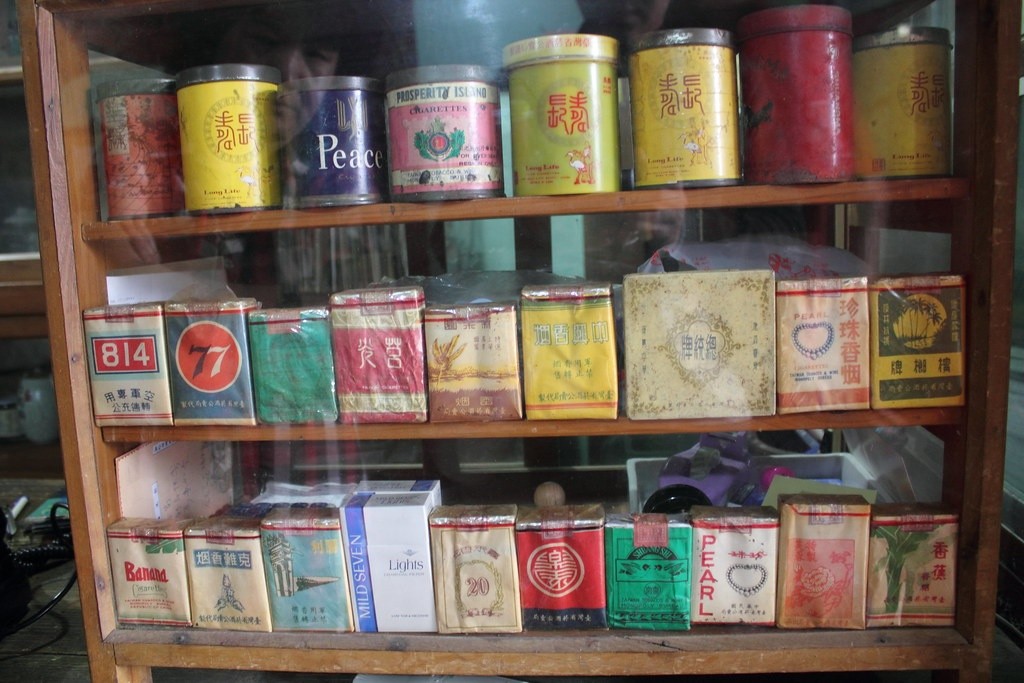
[142, 0, 442, 308]
[570, 0, 805, 281]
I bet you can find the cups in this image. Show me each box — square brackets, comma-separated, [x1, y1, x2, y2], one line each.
[17, 376, 61, 444]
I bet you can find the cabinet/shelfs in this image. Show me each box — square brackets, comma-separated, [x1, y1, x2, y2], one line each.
[15, 0, 1024, 683]
